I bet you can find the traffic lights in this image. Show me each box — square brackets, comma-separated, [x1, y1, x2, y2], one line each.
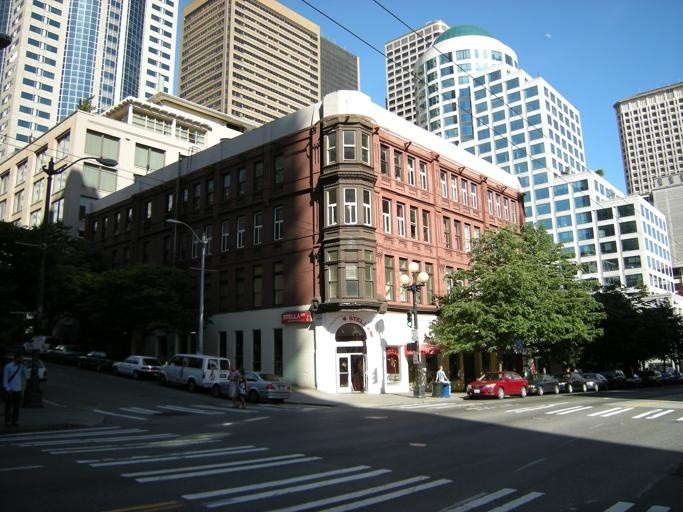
[408, 309, 412, 328]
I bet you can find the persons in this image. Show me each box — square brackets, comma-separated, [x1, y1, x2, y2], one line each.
[237, 366, 248, 408]
[227, 365, 240, 408]
[2, 353, 32, 428]
[435, 365, 449, 383]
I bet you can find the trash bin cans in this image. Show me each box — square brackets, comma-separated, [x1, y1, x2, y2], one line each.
[431, 381, 441, 397]
[440, 382, 452, 398]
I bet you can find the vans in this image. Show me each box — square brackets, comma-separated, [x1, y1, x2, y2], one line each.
[160, 354, 231, 393]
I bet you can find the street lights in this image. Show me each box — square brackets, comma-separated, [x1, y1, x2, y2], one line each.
[27, 157, 118, 404]
[400, 261, 429, 395]
[167, 219, 207, 355]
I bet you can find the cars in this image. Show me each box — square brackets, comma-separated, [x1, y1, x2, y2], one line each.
[554, 373, 587, 393]
[0, 355, 47, 385]
[212, 371, 291, 403]
[602, 368, 683, 387]
[467, 372, 528, 400]
[70, 350, 116, 372]
[525, 374, 561, 395]
[582, 373, 607, 392]
[113, 355, 162, 379]
[45, 344, 82, 366]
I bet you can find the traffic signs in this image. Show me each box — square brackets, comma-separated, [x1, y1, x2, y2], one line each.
[280, 311, 314, 324]
